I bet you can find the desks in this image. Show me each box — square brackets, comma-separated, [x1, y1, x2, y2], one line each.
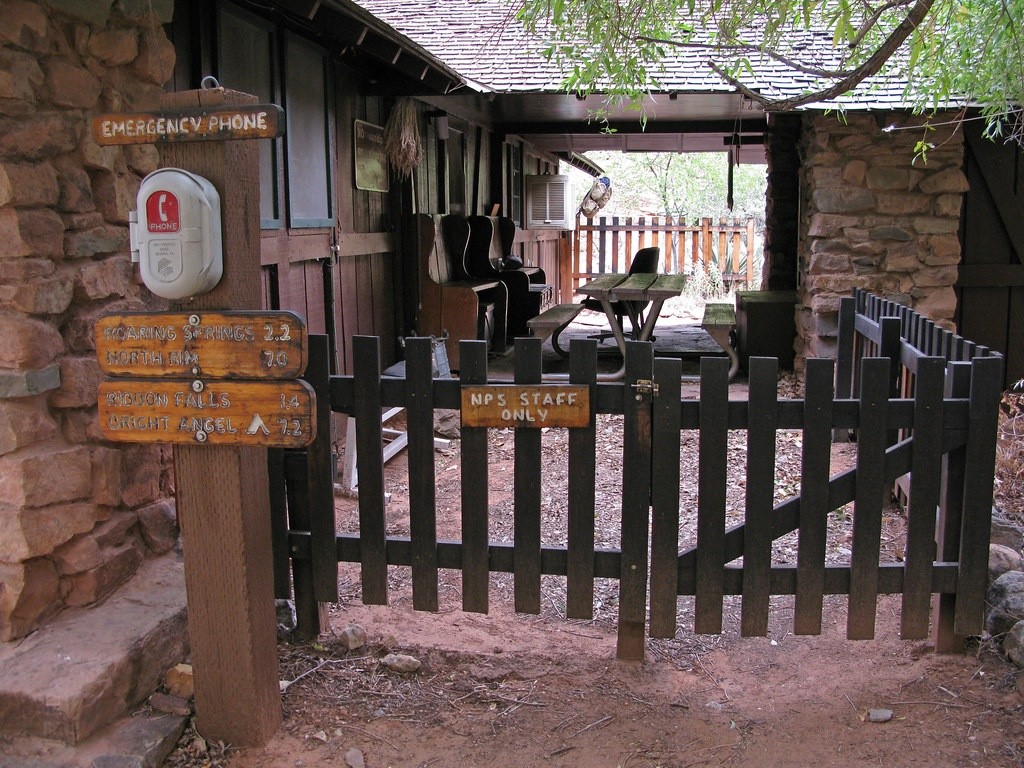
[469, 216, 552, 341]
[576, 273, 687, 381]
[418, 214, 509, 369]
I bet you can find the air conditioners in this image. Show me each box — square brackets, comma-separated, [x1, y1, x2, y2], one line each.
[527, 174, 576, 231]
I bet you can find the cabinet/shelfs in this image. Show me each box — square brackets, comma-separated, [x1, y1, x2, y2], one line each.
[736, 290, 798, 371]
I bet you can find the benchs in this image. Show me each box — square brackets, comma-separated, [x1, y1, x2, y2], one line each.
[527, 304, 585, 381]
[701, 304, 739, 380]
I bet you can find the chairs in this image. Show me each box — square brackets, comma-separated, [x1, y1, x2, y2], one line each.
[580, 247, 660, 344]
